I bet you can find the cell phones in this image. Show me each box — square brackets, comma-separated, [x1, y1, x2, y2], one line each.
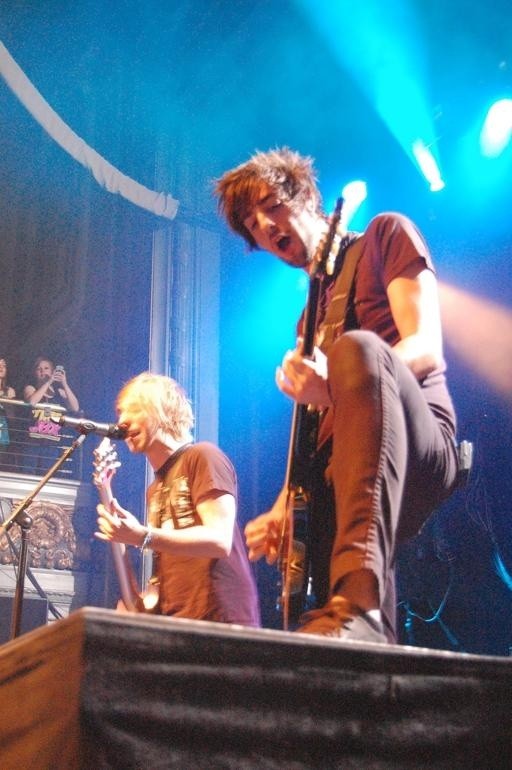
[55, 365, 63, 376]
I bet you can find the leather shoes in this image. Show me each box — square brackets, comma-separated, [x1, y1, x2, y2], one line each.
[290, 603, 388, 645]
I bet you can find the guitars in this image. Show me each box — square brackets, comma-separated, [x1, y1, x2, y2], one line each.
[87, 429, 168, 621]
[274, 188, 346, 630]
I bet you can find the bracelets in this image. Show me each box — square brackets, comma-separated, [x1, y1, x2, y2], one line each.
[139, 527, 151, 552]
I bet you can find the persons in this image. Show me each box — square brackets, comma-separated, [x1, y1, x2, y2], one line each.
[94, 373, 260, 626]
[23, 358, 77, 477]
[217, 147, 457, 643]
[0, 357, 15, 470]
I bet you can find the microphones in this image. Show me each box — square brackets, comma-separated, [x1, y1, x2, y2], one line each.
[50, 416, 128, 440]
